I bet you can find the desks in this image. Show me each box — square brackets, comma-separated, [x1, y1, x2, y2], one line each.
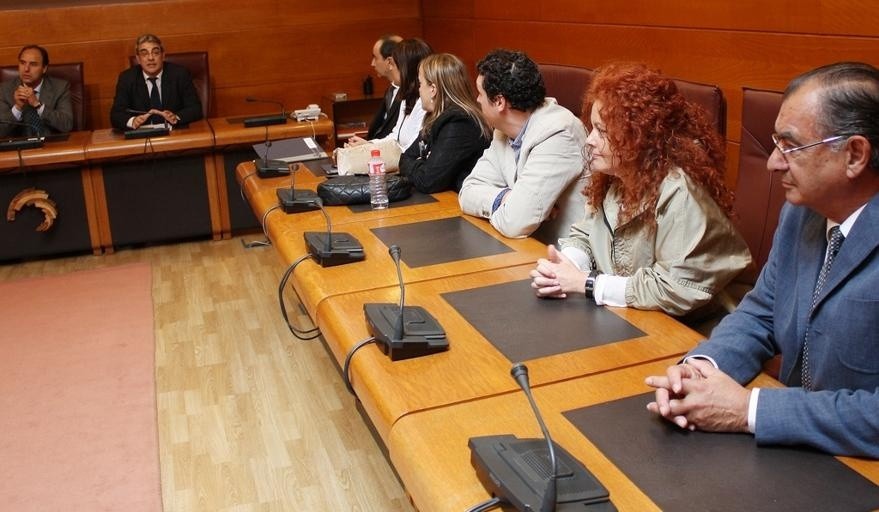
[202, 104, 340, 240]
[236, 146, 879, 512]
[0, 128, 104, 271]
[80, 115, 224, 263]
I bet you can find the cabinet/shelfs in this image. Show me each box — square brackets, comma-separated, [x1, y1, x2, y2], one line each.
[315, 88, 391, 154]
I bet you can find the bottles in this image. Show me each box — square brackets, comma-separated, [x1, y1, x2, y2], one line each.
[368, 149, 389, 211]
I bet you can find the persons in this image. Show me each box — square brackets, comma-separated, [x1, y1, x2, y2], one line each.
[0, 45, 74, 139]
[644, 60, 879, 460]
[111, 33, 204, 131]
[457, 47, 593, 250]
[347, 35, 495, 194]
[529, 62, 759, 339]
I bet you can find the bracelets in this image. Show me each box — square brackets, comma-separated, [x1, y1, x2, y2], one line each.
[586, 271, 605, 298]
[34, 101, 42, 109]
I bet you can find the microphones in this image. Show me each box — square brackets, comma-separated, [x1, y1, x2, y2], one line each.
[303, 196, 366, 268]
[468, 363, 624, 512]
[362, 244, 450, 361]
[0, 119, 42, 151]
[254, 140, 291, 177]
[238, 96, 286, 125]
[124, 106, 170, 139]
[276, 162, 323, 214]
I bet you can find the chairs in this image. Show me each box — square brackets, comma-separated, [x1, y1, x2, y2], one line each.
[662, 77, 728, 174]
[125, 49, 214, 120]
[535, 61, 600, 139]
[0, 61, 88, 133]
[726, 85, 786, 384]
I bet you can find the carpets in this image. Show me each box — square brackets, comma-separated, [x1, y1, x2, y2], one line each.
[0, 259, 173, 512]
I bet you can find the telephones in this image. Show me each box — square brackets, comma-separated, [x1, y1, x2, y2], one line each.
[290, 104, 321, 122]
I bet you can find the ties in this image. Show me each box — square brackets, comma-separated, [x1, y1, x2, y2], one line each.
[28, 90, 45, 138]
[146, 76, 161, 124]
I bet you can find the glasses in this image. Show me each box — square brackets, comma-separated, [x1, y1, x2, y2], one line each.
[770, 132, 850, 164]
